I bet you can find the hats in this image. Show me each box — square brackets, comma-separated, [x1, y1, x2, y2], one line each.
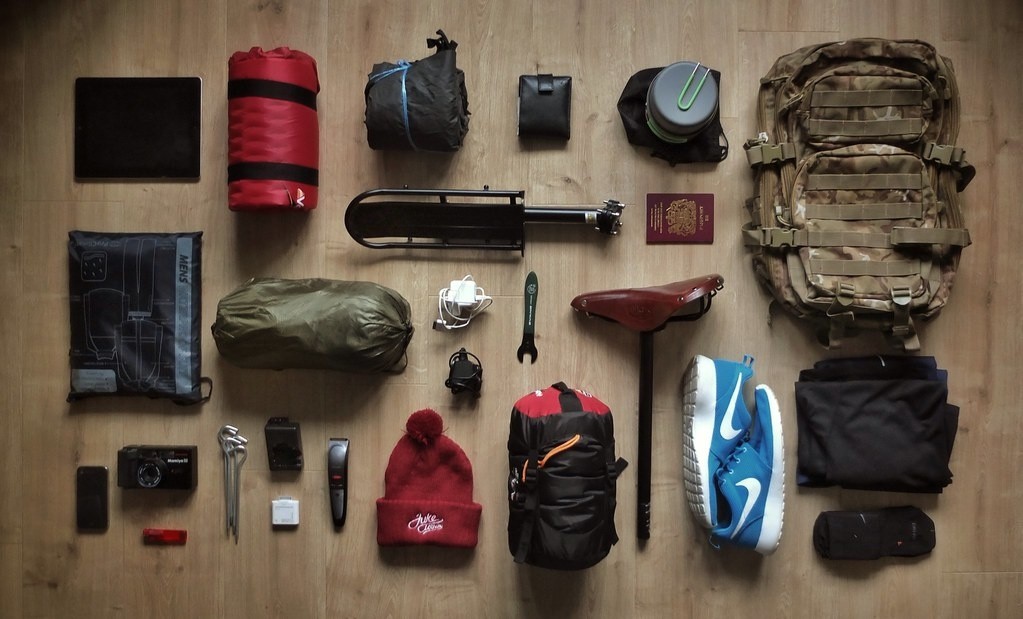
[374, 410, 481, 548]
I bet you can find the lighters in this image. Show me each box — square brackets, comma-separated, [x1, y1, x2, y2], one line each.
[142, 527, 188, 546]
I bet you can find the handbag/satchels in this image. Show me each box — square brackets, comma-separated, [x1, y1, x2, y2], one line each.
[508, 379, 628, 571]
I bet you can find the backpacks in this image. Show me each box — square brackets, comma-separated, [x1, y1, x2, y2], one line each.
[743, 37, 976, 352]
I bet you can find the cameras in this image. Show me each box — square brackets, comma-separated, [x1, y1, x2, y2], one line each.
[117, 444, 196, 490]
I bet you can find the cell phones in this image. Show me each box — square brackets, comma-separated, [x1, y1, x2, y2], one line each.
[77, 464, 109, 533]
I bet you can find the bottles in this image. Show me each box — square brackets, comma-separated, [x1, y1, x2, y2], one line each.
[644, 60, 720, 148]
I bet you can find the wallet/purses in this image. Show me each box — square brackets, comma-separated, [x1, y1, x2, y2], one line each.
[516, 73, 573, 139]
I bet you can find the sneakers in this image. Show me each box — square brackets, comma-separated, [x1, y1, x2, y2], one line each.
[682, 353, 754, 528]
[708, 385, 785, 554]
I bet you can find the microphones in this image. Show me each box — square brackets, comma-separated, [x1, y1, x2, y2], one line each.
[448, 348, 478, 392]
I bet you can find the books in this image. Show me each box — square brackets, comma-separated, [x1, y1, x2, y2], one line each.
[645, 193, 715, 245]
